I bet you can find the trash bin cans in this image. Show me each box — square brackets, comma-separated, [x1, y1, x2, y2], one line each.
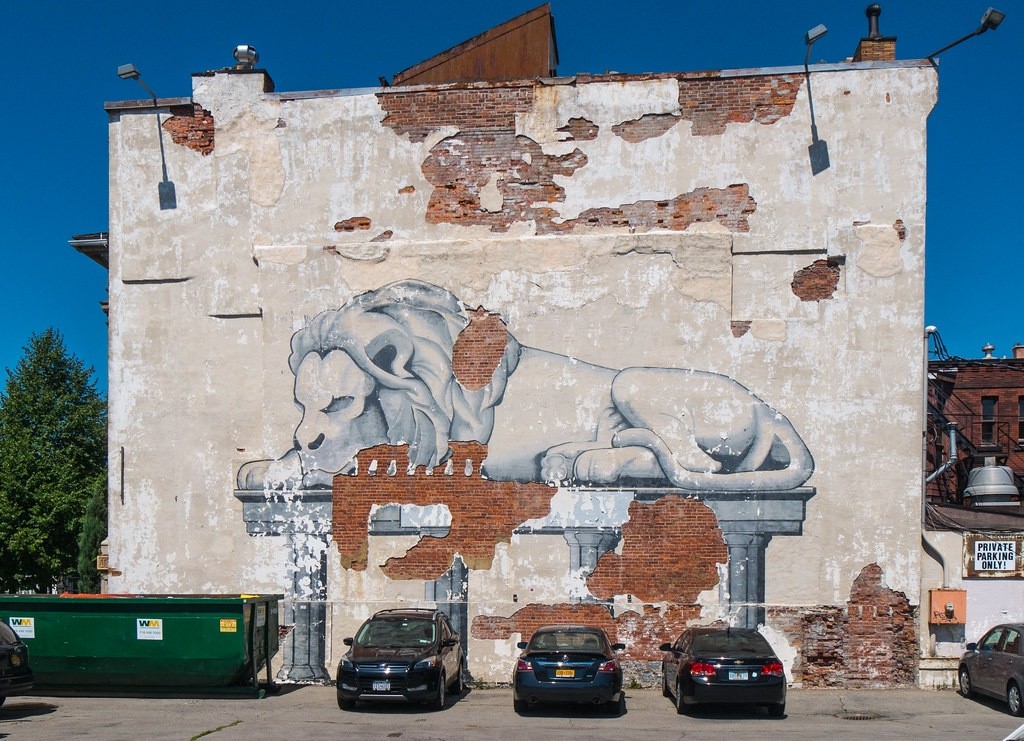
[0, 593, 285, 700]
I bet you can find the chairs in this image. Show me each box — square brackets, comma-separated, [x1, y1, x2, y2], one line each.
[542, 635, 557, 649]
[581, 638, 597, 649]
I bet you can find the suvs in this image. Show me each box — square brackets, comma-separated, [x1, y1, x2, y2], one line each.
[0, 620, 33, 707]
[336, 608, 465, 711]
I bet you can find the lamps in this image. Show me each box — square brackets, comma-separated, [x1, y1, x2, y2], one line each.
[805, 23, 827, 63]
[117, 64, 155, 98]
[925, 7, 1005, 59]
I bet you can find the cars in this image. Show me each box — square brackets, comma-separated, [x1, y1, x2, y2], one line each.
[958, 623, 1024, 716]
[513, 626, 625, 714]
[659, 627, 786, 717]
